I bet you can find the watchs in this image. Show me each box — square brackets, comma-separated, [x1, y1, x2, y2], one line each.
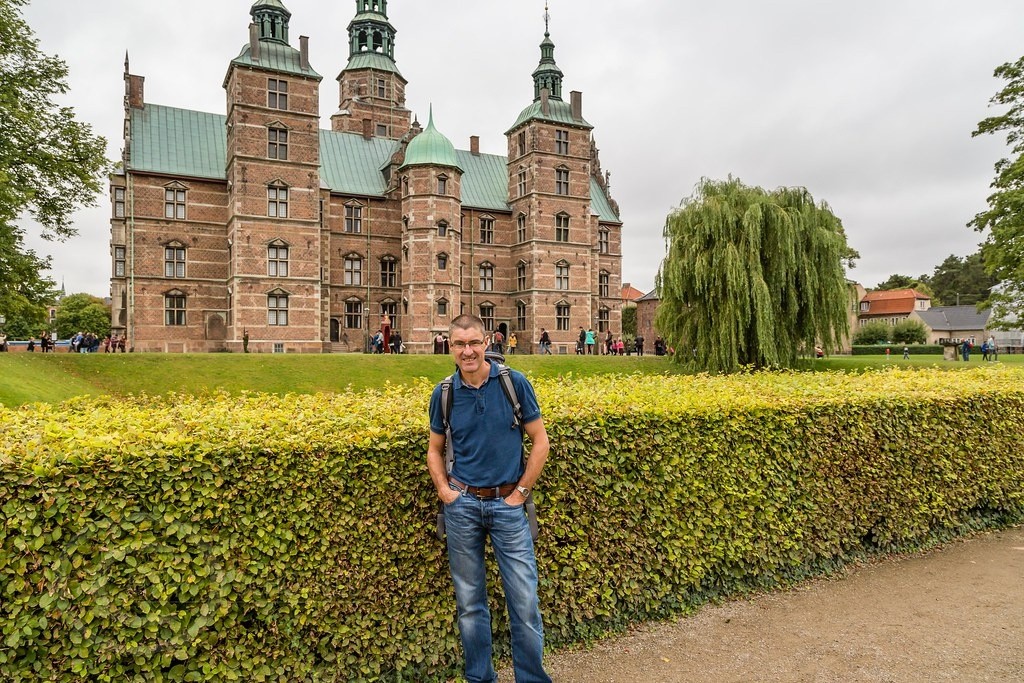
[516, 485, 530, 498]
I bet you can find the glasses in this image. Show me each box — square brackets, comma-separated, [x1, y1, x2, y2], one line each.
[450, 336, 485, 349]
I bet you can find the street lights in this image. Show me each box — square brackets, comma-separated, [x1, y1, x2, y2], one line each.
[363, 306, 370, 354]
[594, 317, 601, 356]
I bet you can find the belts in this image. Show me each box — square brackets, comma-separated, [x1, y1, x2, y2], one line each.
[449, 478, 515, 497]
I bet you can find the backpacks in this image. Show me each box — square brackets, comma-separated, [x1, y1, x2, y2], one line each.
[442, 352, 523, 433]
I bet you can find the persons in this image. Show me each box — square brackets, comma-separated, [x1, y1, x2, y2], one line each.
[243, 330, 249, 354]
[0, 327, 127, 355]
[343, 327, 676, 357]
[417, 317, 558, 683]
[891, 335, 1000, 362]
